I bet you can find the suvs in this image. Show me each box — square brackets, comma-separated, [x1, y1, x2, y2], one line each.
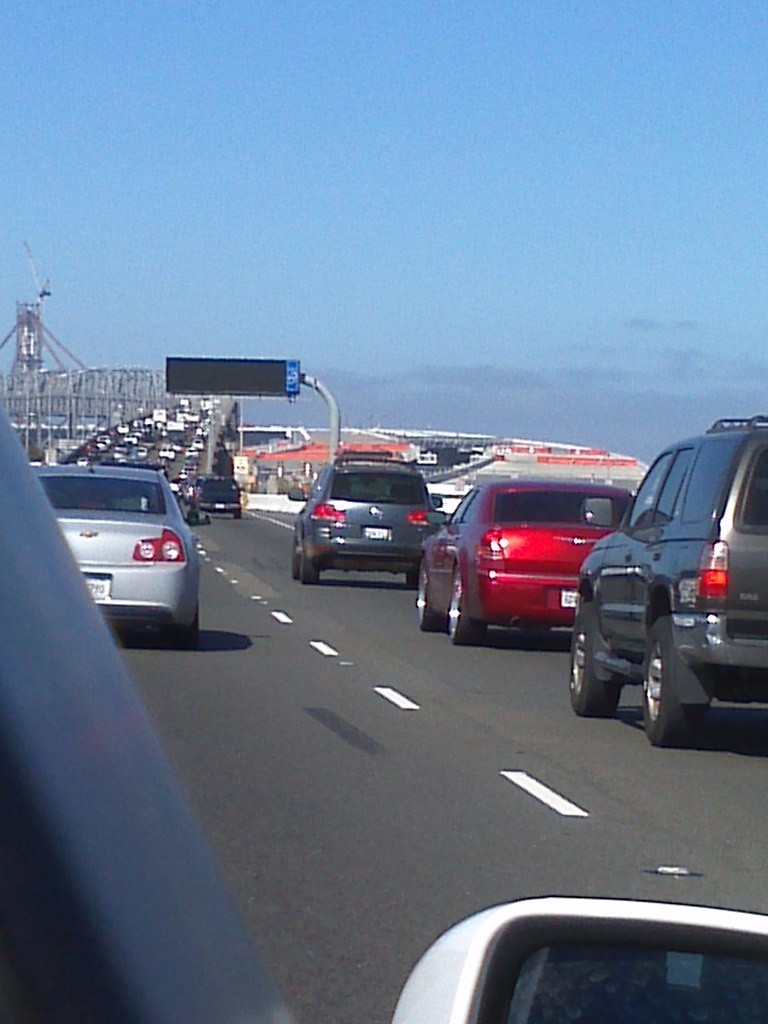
[564, 415, 766, 748]
[280, 457, 436, 590]
[193, 476, 245, 519]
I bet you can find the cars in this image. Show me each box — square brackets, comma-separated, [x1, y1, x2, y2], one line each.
[413, 478, 633, 649]
[70, 391, 220, 510]
[25, 461, 201, 638]
